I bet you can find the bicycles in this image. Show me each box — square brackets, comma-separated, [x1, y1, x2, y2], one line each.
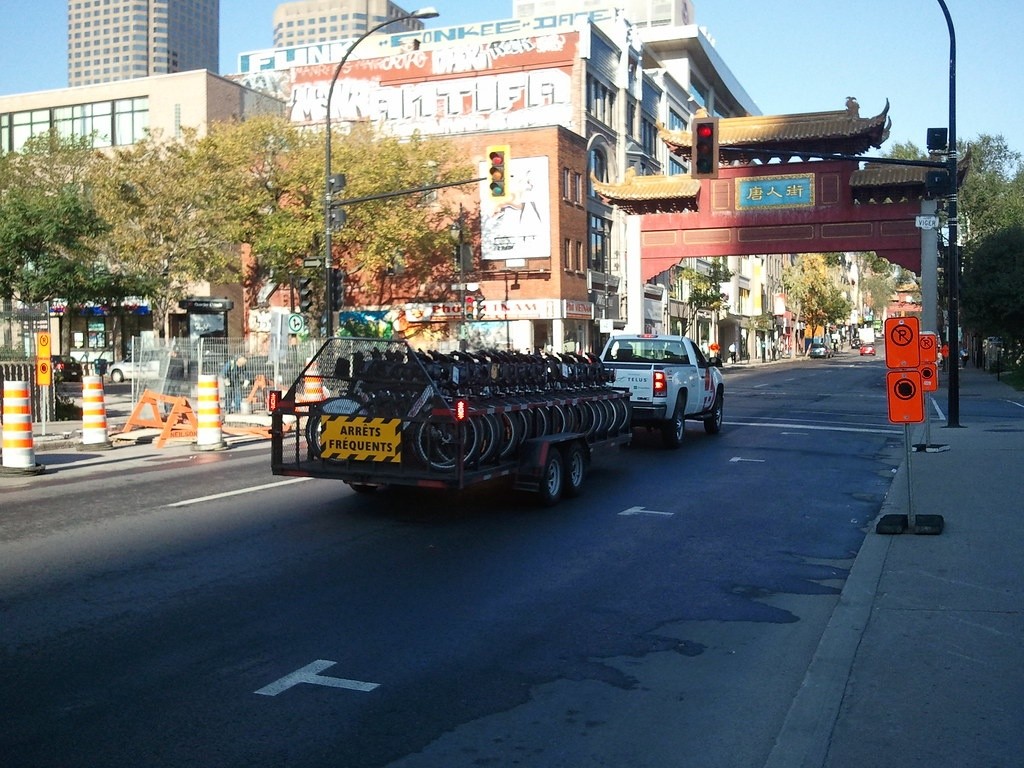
[305, 346, 631, 474]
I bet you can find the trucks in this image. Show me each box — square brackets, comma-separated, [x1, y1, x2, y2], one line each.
[858, 328, 875, 347]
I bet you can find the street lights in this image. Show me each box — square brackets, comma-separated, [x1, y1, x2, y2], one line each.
[449, 202, 466, 355]
[324, 4, 441, 335]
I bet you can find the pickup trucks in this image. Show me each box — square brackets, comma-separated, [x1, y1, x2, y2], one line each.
[601, 334, 725, 449]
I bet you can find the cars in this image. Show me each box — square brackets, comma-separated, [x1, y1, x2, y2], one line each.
[860, 345, 876, 356]
[50, 354, 83, 382]
[851, 338, 862, 349]
[809, 343, 835, 359]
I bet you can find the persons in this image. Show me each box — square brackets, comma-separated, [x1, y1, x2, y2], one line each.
[728, 343, 736, 363]
[159, 345, 183, 414]
[219, 357, 250, 413]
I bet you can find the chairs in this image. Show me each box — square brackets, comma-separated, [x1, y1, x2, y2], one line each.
[661, 345, 685, 363]
[615, 344, 637, 362]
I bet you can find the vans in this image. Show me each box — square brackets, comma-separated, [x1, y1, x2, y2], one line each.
[107, 348, 166, 384]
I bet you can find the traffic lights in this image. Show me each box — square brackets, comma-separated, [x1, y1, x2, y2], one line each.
[476, 296, 485, 321]
[464, 295, 474, 320]
[298, 274, 314, 313]
[328, 269, 344, 314]
[691, 116, 718, 180]
[486, 144, 511, 201]
